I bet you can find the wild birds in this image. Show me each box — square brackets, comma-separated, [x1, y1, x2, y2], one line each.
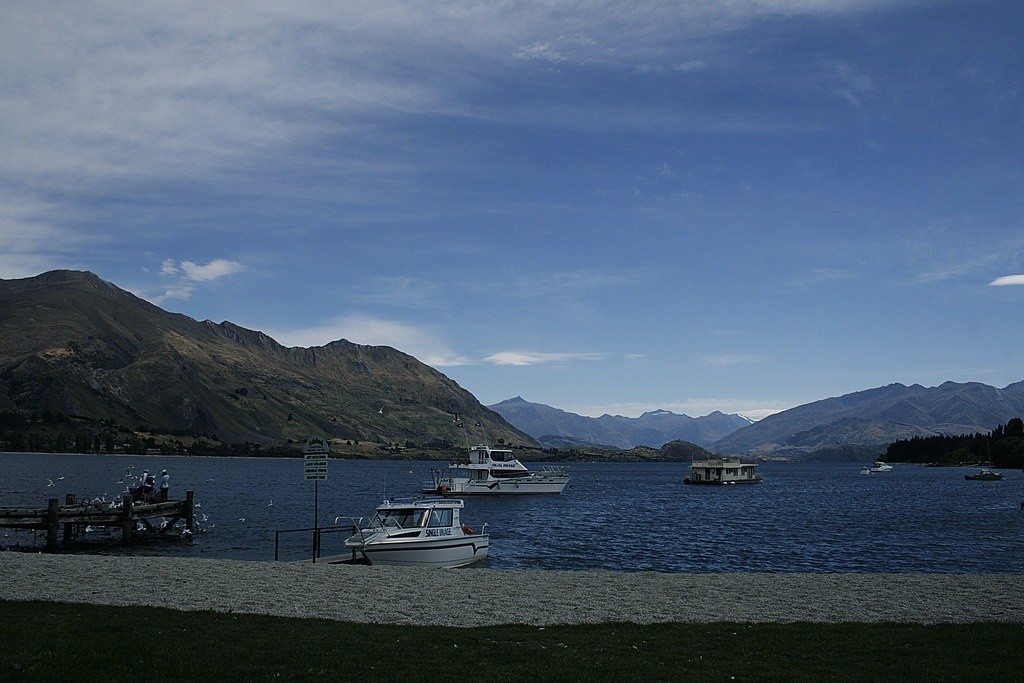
[239, 517, 245, 523]
[4, 526, 35, 538]
[47, 477, 65, 487]
[106, 464, 142, 486]
[376, 404, 386, 416]
[161, 499, 216, 534]
[142, 469, 169, 489]
[268, 500, 273, 507]
[85, 487, 146, 532]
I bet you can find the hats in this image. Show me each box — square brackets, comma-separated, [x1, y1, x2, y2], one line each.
[161, 469, 166, 473]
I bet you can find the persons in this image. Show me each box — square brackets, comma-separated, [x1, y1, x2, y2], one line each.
[159, 469, 170, 502]
[142, 470, 156, 503]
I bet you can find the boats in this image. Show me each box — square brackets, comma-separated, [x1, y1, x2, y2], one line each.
[344, 497, 491, 569]
[682, 459, 764, 484]
[420, 414, 572, 496]
[871, 461, 894, 472]
[963, 470, 1005, 480]
[860, 467, 872, 475]
